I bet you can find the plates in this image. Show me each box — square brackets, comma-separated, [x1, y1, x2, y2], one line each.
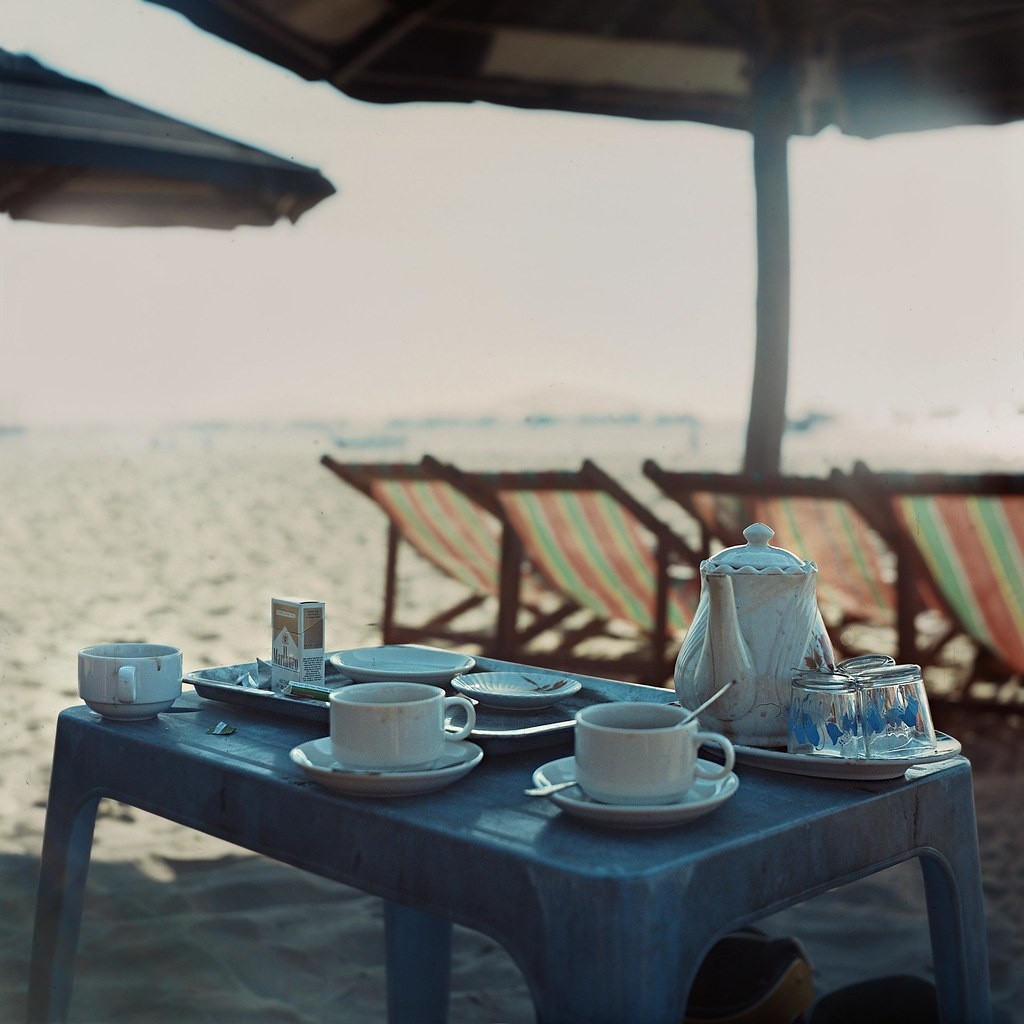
[532, 756, 738, 830]
[329, 648, 475, 685]
[451, 672, 581, 710]
[289, 737, 483, 797]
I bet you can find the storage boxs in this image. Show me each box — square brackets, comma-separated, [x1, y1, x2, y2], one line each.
[270, 596, 326, 687]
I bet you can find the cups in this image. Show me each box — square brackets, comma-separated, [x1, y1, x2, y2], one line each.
[855, 664, 937, 759]
[78, 643, 182, 720]
[576, 701, 735, 806]
[329, 682, 476, 771]
[836, 655, 896, 759]
[786, 672, 858, 759]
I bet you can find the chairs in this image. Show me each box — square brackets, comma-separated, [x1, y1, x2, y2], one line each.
[852, 458, 1024, 719]
[641, 457, 966, 713]
[319, 452, 640, 649]
[442, 458, 702, 685]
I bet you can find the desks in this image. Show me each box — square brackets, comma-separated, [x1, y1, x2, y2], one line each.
[27, 648, 995, 1024]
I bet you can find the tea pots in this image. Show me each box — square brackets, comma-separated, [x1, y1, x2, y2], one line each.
[675, 522, 837, 747]
